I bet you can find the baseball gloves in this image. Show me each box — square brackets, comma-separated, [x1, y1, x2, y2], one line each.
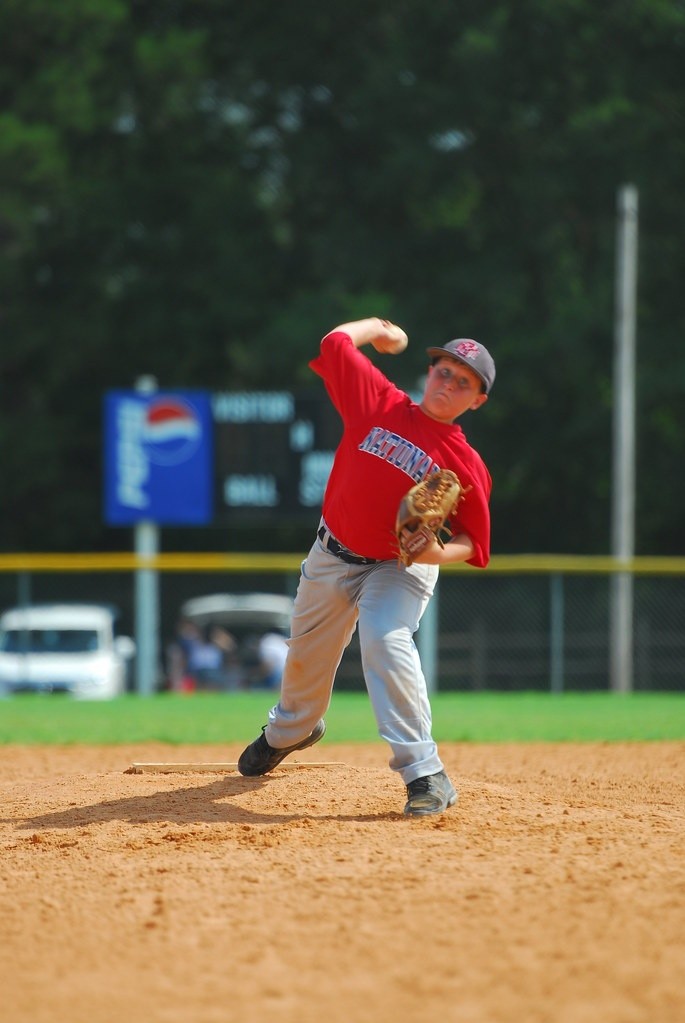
[395, 469, 462, 567]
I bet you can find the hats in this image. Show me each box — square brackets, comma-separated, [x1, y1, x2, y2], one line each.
[426, 338, 496, 394]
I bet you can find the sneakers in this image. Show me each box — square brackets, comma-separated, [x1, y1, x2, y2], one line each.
[238, 718, 325, 776]
[404, 769, 458, 815]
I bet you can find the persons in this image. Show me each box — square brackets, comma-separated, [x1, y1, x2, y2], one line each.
[167, 624, 288, 691]
[238, 317, 496, 816]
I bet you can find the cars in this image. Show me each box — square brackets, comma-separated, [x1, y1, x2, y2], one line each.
[0, 601, 137, 703]
[164, 589, 298, 693]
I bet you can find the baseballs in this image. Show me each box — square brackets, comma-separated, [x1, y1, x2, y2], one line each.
[384, 326, 409, 356]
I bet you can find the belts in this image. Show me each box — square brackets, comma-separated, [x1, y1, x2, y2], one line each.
[318, 525, 376, 565]
